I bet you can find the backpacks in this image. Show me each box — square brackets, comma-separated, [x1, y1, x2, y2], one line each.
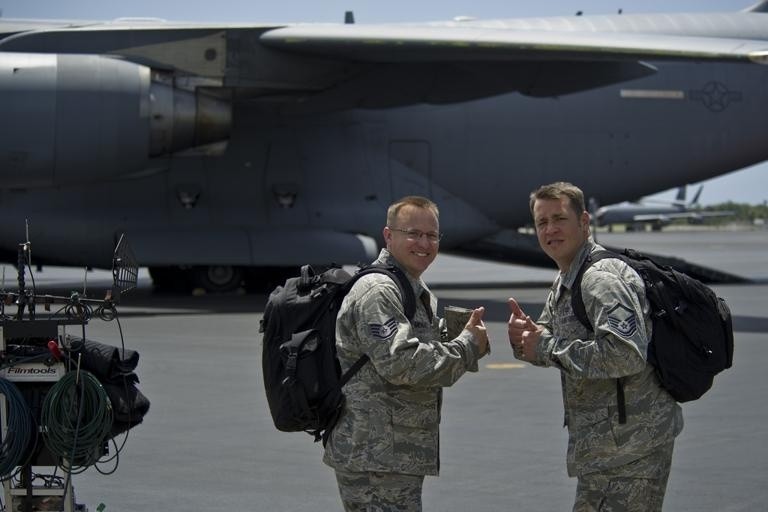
[571, 249, 733, 403]
[259, 262, 416, 432]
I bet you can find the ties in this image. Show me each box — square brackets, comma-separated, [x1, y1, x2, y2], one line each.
[390, 227, 444, 244]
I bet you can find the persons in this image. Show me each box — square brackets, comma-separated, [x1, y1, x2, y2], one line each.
[507, 181, 685, 512]
[321, 196, 489, 512]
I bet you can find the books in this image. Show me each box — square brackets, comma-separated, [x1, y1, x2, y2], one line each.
[444, 305, 479, 373]
